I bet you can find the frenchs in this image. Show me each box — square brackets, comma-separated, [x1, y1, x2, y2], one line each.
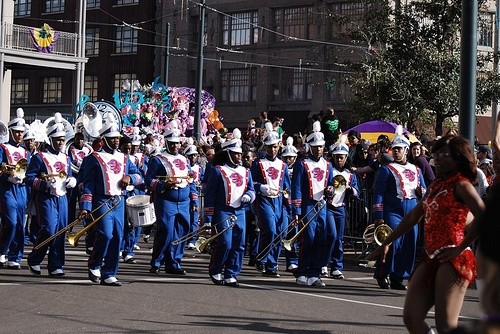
[80, 102, 123, 144]
[62, 102, 103, 174]
[43, 116, 75, 151]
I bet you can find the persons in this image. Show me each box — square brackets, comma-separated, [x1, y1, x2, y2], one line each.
[476, 145, 495, 178]
[444, 125, 457, 137]
[0, 102, 448, 290]
[474, 167, 489, 198]
[367, 135, 486, 334]
[434, 130, 442, 142]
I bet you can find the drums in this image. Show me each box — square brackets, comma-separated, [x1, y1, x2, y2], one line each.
[125, 194, 157, 227]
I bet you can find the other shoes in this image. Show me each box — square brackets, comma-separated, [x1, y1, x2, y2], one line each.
[8, 261, 20, 269]
[0, 255, 6, 265]
[88, 268, 101, 282]
[29, 265, 41, 276]
[118, 235, 407, 291]
[49, 269, 65, 277]
[100, 277, 121, 286]
[86, 247, 93, 254]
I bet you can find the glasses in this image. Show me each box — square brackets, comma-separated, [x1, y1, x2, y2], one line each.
[431, 152, 451, 158]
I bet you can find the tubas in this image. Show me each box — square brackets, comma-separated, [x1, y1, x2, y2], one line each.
[0, 157, 29, 180]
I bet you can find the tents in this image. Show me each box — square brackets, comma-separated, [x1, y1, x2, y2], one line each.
[342, 120, 419, 146]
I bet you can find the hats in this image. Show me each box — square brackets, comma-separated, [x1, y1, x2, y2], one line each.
[185, 137, 198, 155]
[282, 136, 298, 157]
[263, 122, 280, 145]
[330, 135, 349, 155]
[163, 121, 180, 143]
[221, 128, 242, 153]
[99, 113, 123, 139]
[23, 124, 35, 140]
[47, 112, 66, 140]
[306, 120, 325, 146]
[391, 124, 410, 150]
[8, 108, 26, 131]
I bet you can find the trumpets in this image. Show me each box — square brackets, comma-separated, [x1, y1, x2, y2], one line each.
[36, 170, 67, 184]
[155, 173, 194, 183]
[333, 174, 346, 189]
[267, 186, 291, 199]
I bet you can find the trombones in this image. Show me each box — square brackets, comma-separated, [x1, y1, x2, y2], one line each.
[34, 195, 122, 251]
[256, 195, 326, 262]
[171, 214, 238, 252]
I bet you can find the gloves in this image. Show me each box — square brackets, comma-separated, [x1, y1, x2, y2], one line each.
[65, 177, 76, 189]
[47, 181, 56, 189]
[260, 184, 271, 196]
[241, 195, 251, 203]
